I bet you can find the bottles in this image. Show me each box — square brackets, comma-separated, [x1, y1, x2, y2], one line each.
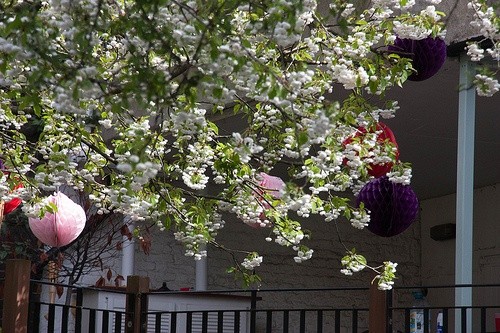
[410, 294, 431, 333]
[436, 311, 443, 333]
